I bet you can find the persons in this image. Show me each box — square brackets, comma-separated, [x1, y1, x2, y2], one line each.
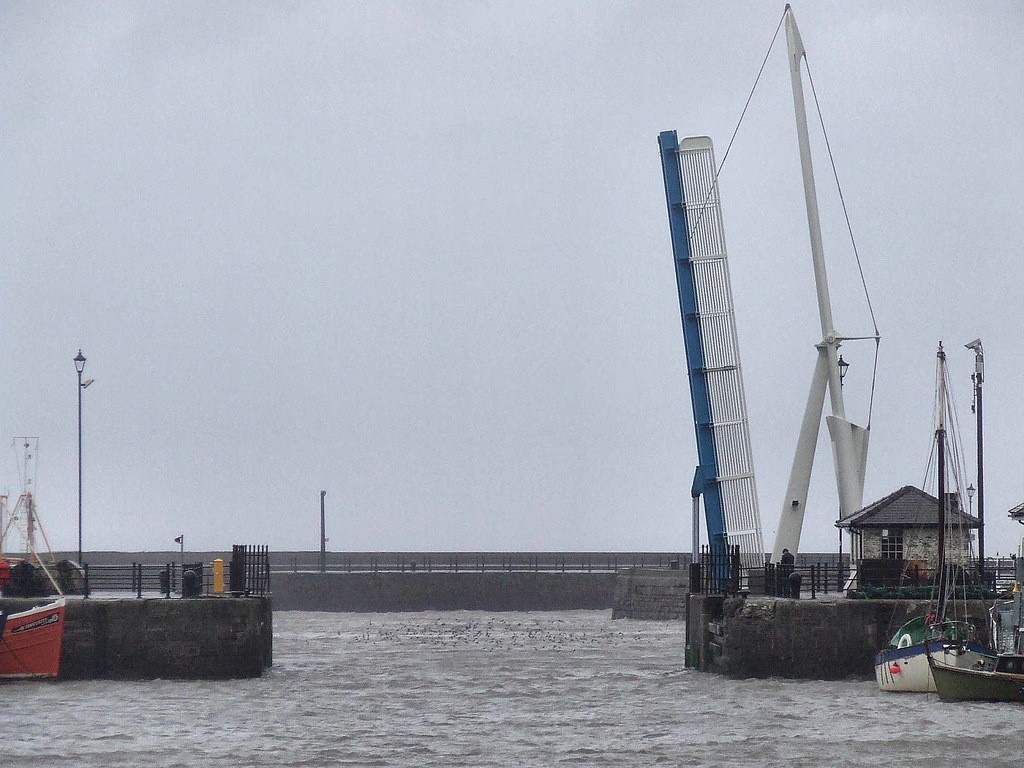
[780, 549, 794, 598]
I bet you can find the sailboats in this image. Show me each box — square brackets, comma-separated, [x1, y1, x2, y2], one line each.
[874, 339, 1000, 693]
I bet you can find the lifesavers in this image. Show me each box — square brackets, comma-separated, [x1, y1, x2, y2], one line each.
[897, 634, 913, 649]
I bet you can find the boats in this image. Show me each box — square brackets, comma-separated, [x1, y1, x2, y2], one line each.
[926, 646, 1024, 704]
[0, 492, 66, 679]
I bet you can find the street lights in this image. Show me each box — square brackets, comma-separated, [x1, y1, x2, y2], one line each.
[966, 484, 977, 570]
[73, 349, 86, 564]
